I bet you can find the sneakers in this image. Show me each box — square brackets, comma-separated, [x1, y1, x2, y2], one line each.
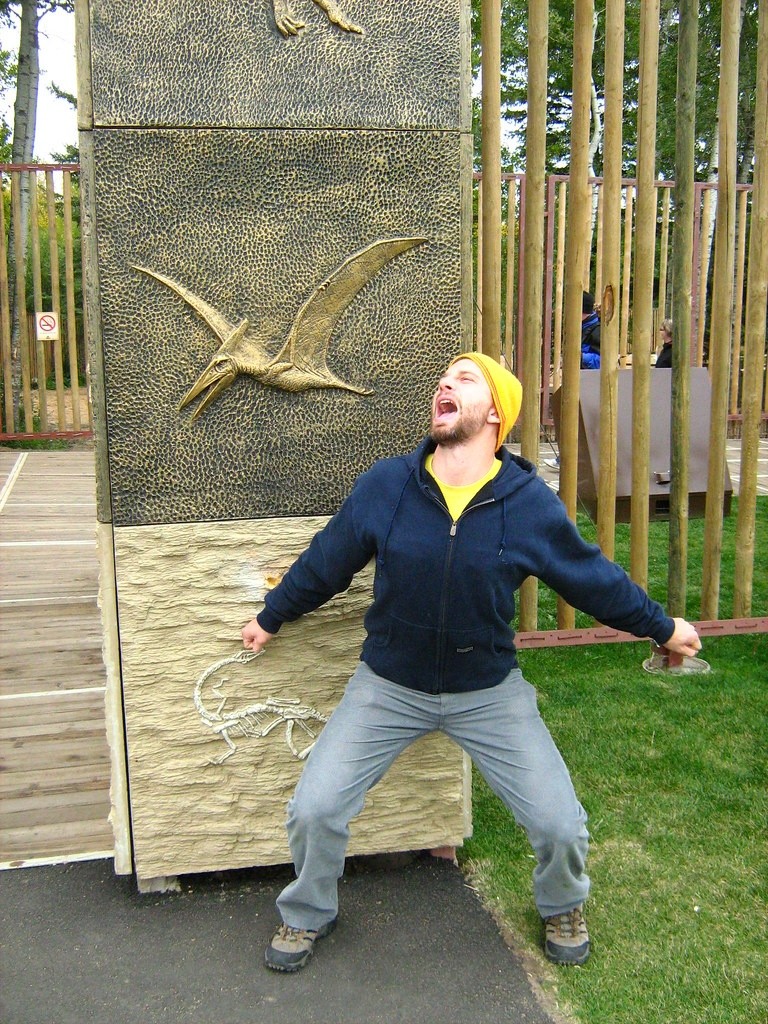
[543, 904, 590, 965]
[264, 914, 337, 972]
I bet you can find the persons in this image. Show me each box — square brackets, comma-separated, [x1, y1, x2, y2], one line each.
[654, 318, 674, 369]
[241, 350, 703, 973]
[543, 290, 601, 470]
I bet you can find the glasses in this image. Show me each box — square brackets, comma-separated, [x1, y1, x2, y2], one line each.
[659, 328, 664, 331]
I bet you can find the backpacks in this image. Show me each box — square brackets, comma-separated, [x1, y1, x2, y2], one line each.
[587, 320, 602, 354]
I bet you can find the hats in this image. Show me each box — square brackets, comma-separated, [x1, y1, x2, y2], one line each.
[449, 352, 523, 453]
[583, 290, 595, 314]
